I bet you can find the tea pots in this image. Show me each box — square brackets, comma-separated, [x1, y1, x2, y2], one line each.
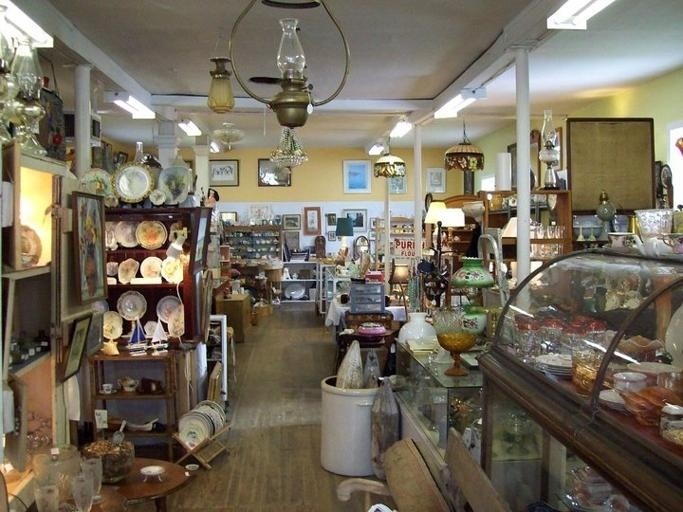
[631, 234, 672, 259]
[118, 376, 141, 394]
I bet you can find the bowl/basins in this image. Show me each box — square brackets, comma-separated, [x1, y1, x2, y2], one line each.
[610, 372, 648, 392]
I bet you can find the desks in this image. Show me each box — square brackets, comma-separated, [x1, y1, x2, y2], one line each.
[66, 457, 190, 512]
[324, 297, 406, 339]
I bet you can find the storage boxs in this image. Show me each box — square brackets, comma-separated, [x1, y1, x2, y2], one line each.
[349, 283, 385, 314]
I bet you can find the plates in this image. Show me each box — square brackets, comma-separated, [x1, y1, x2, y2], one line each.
[104, 220, 169, 249]
[106, 256, 184, 285]
[178, 401, 227, 451]
[148, 187, 166, 204]
[157, 163, 192, 204]
[596, 388, 626, 412]
[284, 283, 305, 299]
[110, 161, 151, 201]
[99, 390, 118, 394]
[21, 226, 44, 266]
[76, 169, 112, 202]
[103, 289, 182, 343]
[533, 352, 573, 381]
[628, 360, 682, 378]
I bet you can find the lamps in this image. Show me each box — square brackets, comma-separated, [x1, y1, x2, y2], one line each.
[387, 264, 409, 305]
[440, 208, 465, 249]
[442, 108, 484, 172]
[270, 126, 307, 169]
[210, 122, 245, 145]
[433, 87, 486, 122]
[101, 91, 156, 121]
[227, 0, 350, 129]
[423, 202, 447, 251]
[374, 132, 406, 179]
[207, 27, 234, 114]
[1, 1, 54, 51]
[546, 1, 613, 30]
[176, 119, 202, 138]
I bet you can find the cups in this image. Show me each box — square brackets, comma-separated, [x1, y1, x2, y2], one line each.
[102, 384, 113, 393]
[30, 450, 103, 512]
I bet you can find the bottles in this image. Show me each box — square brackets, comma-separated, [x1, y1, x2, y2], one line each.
[656, 403, 683, 438]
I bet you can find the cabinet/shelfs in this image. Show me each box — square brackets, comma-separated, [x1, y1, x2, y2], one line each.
[215, 290, 251, 344]
[220, 226, 282, 287]
[443, 195, 480, 254]
[375, 216, 413, 259]
[94, 206, 213, 346]
[480, 191, 573, 265]
[279, 261, 319, 303]
[475, 248, 683, 510]
[0, 150, 73, 512]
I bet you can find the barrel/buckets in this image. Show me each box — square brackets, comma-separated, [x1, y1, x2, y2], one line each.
[264, 268, 283, 282]
[320, 375, 382, 478]
[36, 51, 67, 163]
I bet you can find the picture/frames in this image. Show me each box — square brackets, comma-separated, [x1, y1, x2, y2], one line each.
[56, 313, 91, 382]
[208, 160, 239, 187]
[86, 346, 180, 462]
[71, 190, 108, 306]
[281, 214, 301, 230]
[424, 167, 446, 194]
[325, 208, 384, 256]
[303, 207, 321, 236]
[91, 139, 194, 196]
[341, 158, 372, 194]
[388, 174, 407, 195]
[256, 158, 292, 187]
[506, 126, 564, 189]
